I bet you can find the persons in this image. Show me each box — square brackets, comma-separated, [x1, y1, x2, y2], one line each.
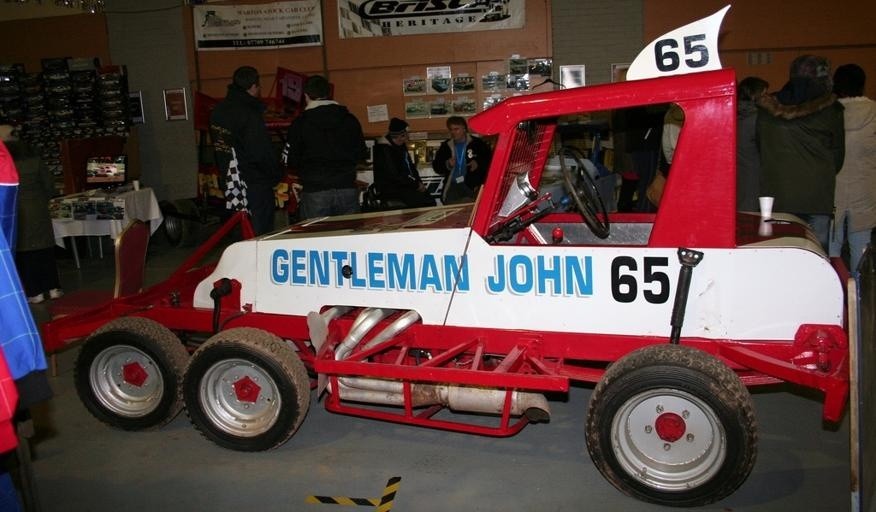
[432, 116, 492, 205]
[208, 66, 284, 244]
[611, 103, 684, 213]
[833, 65, 876, 300]
[755, 55, 845, 258]
[0, 125, 63, 303]
[280, 76, 367, 220]
[372, 118, 437, 208]
[737, 77, 773, 212]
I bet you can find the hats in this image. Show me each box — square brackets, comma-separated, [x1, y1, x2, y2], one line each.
[388, 117, 408, 138]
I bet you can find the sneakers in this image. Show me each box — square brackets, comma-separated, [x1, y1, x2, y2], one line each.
[49, 288, 65, 300]
[27, 293, 45, 304]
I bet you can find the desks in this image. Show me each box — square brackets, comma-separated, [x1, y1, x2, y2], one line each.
[49, 184, 163, 269]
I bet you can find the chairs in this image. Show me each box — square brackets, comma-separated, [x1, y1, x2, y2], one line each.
[362, 143, 409, 209]
[49, 219, 152, 377]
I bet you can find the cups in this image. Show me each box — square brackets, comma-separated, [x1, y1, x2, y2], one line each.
[758, 196, 775, 215]
[758, 217, 772, 237]
[133, 180, 139, 191]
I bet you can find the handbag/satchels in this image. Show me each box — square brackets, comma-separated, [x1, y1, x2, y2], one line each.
[648, 171, 667, 208]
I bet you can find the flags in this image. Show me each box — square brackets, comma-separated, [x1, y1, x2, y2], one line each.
[224, 147, 248, 212]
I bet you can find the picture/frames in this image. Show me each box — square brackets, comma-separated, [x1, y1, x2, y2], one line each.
[130, 90, 144, 125]
[163, 88, 189, 121]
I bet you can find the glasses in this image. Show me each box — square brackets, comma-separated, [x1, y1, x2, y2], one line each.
[400, 132, 407, 137]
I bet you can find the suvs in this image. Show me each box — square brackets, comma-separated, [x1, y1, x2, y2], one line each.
[38, 1, 849, 507]
